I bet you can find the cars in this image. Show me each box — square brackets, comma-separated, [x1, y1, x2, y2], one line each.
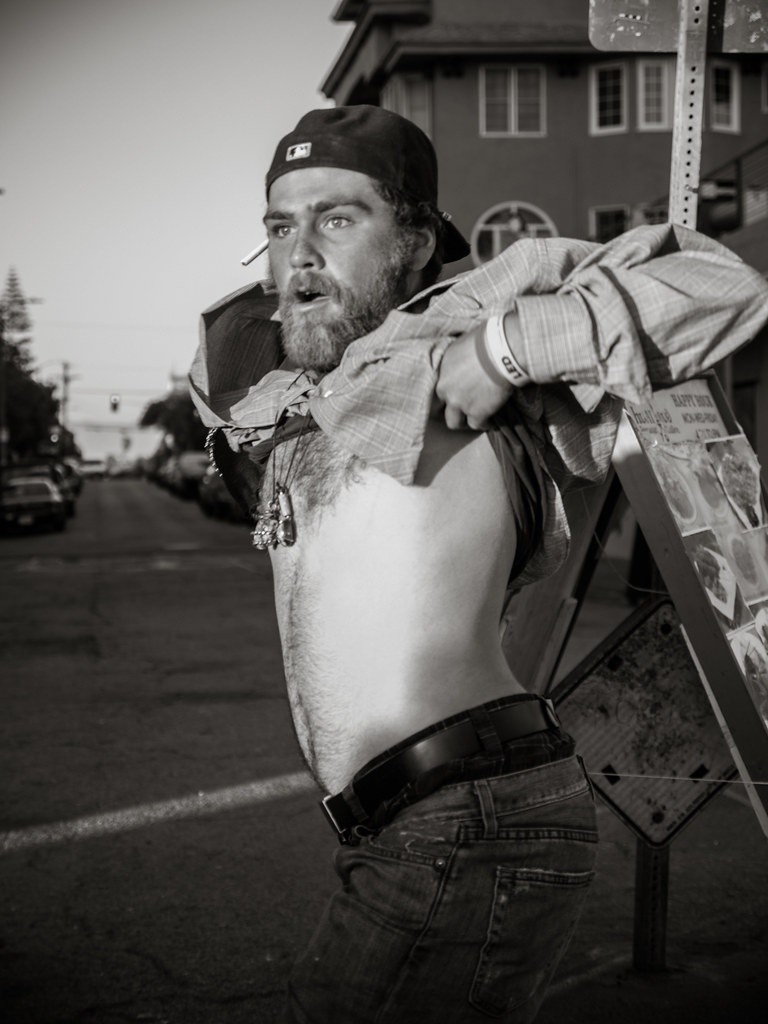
[31, 466, 77, 516]
[166, 452, 212, 494]
[202, 461, 243, 516]
[0, 476, 66, 532]
[60, 459, 82, 495]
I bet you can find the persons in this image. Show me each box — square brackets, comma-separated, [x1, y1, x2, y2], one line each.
[192, 100, 767, 1022]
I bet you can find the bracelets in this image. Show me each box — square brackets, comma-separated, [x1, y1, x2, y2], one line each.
[473, 312, 532, 393]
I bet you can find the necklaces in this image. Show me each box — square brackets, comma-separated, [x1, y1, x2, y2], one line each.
[250, 406, 310, 550]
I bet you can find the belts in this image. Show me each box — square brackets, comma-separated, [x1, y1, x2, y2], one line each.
[320, 695, 563, 844]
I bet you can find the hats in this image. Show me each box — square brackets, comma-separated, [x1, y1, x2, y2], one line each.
[264, 104, 473, 268]
